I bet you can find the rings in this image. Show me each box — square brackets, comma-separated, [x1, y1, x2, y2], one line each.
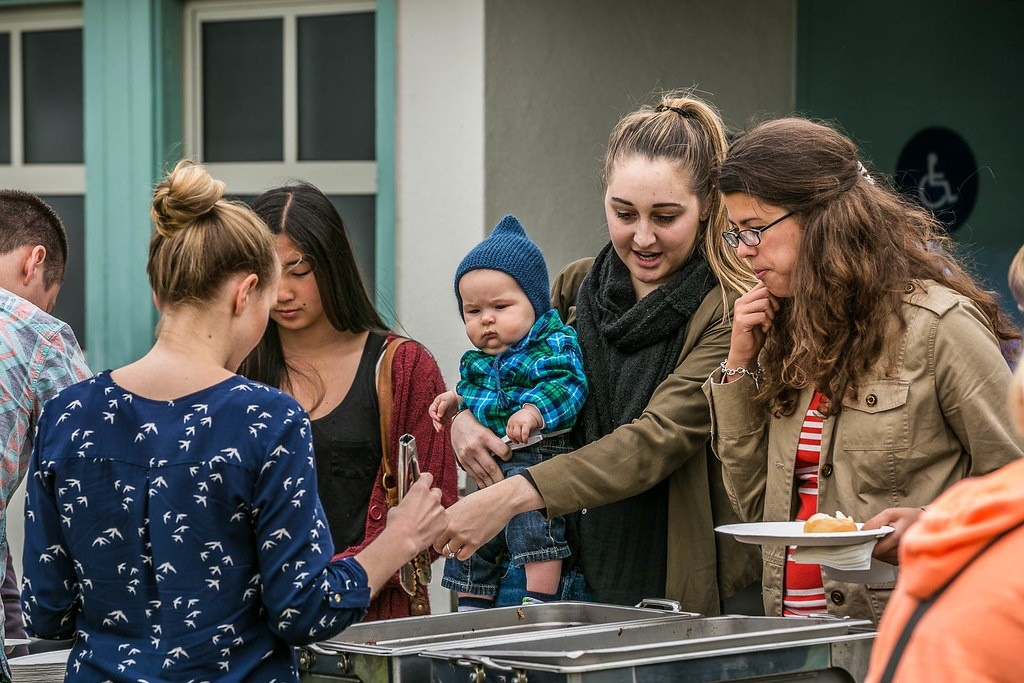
[447, 544, 462, 558]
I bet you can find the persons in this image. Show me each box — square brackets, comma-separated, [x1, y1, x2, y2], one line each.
[18, 167, 449, 683]
[0, 187, 96, 683]
[241, 184, 457, 621]
[434, 99, 760, 613]
[862, 247, 1024, 682]
[427, 215, 589, 615]
[703, 118, 1024, 640]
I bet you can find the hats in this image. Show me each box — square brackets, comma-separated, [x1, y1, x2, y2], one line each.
[453, 214, 550, 323]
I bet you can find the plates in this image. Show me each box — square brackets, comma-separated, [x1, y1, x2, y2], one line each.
[714, 520, 896, 546]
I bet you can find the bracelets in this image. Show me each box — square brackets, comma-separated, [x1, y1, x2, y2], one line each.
[720, 358, 759, 390]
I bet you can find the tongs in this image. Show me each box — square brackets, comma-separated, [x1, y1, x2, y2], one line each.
[397, 433, 432, 597]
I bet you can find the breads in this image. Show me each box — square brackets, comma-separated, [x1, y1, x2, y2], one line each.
[804, 510, 858, 533]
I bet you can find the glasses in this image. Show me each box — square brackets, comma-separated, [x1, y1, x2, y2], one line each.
[722, 210, 796, 249]
